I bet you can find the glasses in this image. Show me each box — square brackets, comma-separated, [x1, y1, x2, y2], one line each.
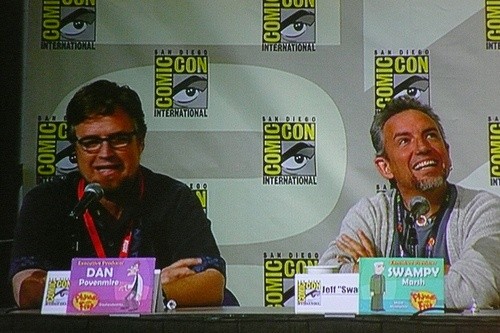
[68, 127, 146, 153]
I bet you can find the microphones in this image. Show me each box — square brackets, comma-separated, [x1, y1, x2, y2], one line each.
[405, 196, 431, 227]
[67, 182, 105, 223]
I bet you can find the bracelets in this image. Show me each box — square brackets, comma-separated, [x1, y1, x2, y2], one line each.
[162, 288, 168, 311]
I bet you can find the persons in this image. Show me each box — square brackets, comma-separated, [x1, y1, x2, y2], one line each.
[317, 95, 500, 312]
[5, 79, 226, 309]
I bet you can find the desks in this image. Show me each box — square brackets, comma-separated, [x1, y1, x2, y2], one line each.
[0, 306, 500, 333]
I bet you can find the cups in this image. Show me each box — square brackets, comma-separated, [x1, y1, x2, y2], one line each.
[304, 265, 340, 274]
